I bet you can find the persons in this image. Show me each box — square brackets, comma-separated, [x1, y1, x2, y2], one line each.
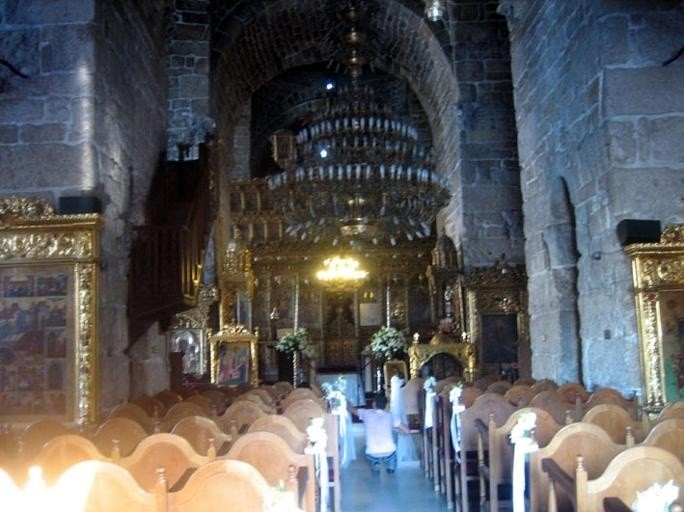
[343, 392, 412, 478]
[389, 366, 410, 433]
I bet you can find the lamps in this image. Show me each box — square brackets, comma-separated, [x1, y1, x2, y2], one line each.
[266, 0, 451, 245]
[314, 245, 368, 280]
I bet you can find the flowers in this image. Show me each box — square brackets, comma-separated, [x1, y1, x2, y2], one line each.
[276, 327, 308, 353]
[369, 325, 408, 357]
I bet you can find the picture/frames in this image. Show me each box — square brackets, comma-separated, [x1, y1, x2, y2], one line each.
[623, 222, 683, 408]
[464, 258, 529, 376]
[406, 331, 476, 384]
[0, 195, 101, 431]
[207, 324, 259, 387]
[382, 358, 408, 409]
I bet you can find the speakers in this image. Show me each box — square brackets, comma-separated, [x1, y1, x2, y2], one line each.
[60, 196, 102, 214]
[618, 219, 661, 245]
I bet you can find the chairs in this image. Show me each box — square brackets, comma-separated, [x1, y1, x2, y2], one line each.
[394, 371, 683, 511]
[0, 381, 348, 511]
